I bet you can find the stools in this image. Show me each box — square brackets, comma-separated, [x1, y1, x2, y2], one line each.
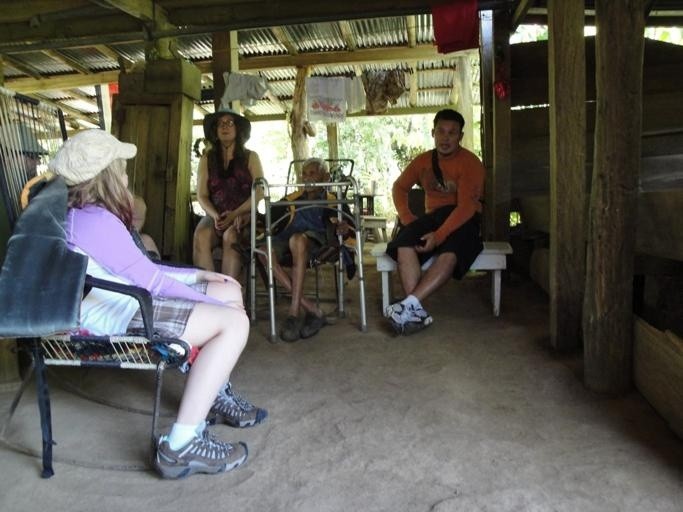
[360, 215, 388, 247]
[370, 242, 514, 317]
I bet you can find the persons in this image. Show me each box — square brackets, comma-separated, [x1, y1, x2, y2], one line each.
[193, 110, 265, 279]
[47, 128, 268, 480]
[385, 109, 485, 336]
[233, 157, 357, 342]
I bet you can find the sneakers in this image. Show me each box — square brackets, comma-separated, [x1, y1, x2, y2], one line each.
[206, 380, 268, 427]
[152, 429, 248, 479]
[281, 309, 328, 342]
[386, 303, 434, 335]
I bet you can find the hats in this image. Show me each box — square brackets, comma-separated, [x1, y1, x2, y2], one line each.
[203, 109, 251, 143]
[48, 128, 138, 187]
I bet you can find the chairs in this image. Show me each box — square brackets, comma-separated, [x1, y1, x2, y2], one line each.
[20, 169, 200, 480]
[217, 238, 269, 311]
[252, 158, 355, 317]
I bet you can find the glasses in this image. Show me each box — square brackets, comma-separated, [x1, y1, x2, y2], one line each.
[216, 119, 235, 127]
